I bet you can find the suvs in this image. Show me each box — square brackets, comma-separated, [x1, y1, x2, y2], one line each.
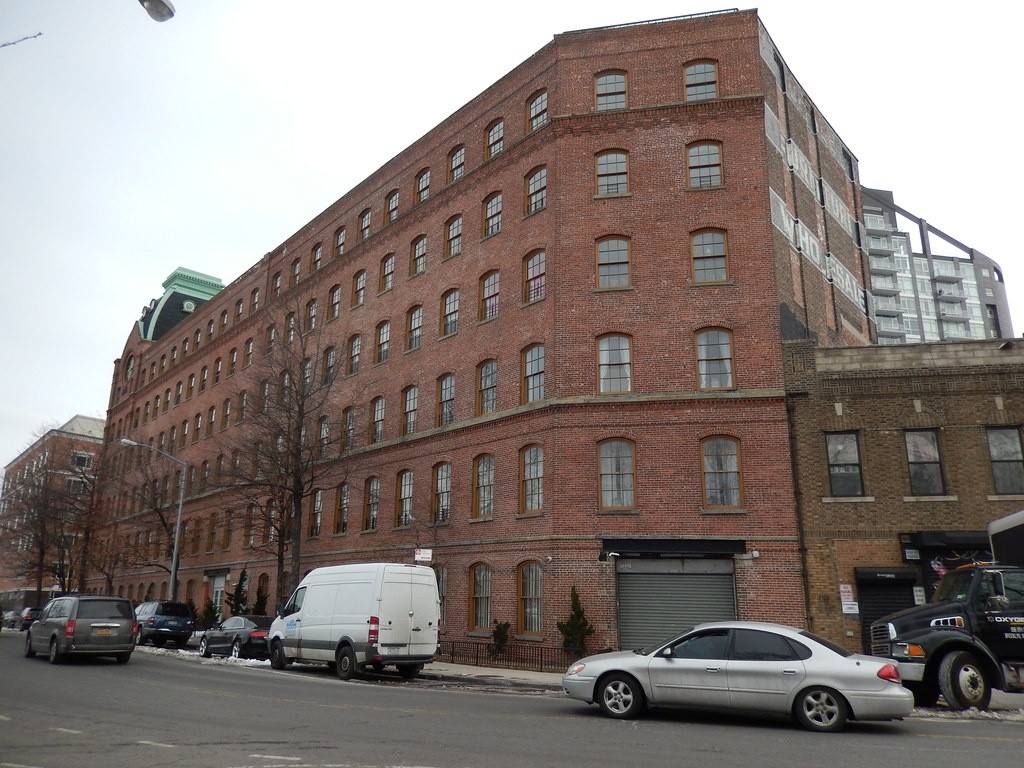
[25, 595, 137, 664]
[134, 599, 196, 647]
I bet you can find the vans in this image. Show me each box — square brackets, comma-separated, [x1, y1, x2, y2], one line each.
[268, 562, 442, 681]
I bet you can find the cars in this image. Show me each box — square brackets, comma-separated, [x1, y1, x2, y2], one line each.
[0, 610, 20, 631]
[18, 607, 43, 632]
[562, 621, 915, 734]
[200, 615, 275, 660]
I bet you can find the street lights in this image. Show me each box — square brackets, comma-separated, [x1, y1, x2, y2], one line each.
[121, 439, 188, 599]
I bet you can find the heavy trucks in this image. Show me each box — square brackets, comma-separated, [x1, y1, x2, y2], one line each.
[870, 509, 1024, 711]
